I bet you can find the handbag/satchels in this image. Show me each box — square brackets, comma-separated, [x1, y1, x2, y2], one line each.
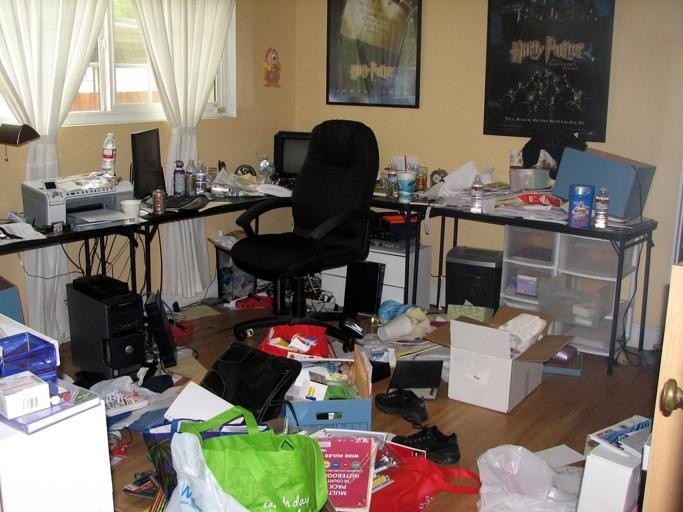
[142, 406, 328, 512]
[370, 444, 481, 512]
[261, 324, 337, 360]
[201, 340, 302, 424]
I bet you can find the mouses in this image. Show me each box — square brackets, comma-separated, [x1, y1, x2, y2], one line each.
[202, 192, 216, 201]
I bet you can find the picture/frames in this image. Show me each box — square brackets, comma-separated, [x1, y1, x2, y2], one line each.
[325, 1, 423, 109]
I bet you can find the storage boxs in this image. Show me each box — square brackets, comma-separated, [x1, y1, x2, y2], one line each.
[420, 218, 635, 415]
[545, 136, 657, 222]
[572, 411, 653, 512]
[273, 341, 380, 435]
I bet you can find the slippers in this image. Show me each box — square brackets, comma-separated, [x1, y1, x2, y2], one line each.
[236, 294, 272, 309]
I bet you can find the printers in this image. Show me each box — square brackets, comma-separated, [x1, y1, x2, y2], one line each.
[22, 172, 134, 232]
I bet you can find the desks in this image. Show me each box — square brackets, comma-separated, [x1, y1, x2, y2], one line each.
[413, 183, 659, 377]
[1, 199, 148, 301]
[262, 173, 412, 309]
[142, 187, 262, 295]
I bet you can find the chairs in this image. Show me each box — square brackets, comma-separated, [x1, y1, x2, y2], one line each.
[217, 118, 384, 352]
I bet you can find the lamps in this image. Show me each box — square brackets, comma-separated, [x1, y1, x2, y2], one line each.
[0, 119, 40, 166]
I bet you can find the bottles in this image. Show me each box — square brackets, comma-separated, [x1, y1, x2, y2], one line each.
[259, 154, 271, 176]
[386, 168, 398, 200]
[593, 187, 611, 229]
[101, 130, 117, 178]
[185, 160, 197, 197]
[467, 174, 483, 214]
[194, 160, 207, 197]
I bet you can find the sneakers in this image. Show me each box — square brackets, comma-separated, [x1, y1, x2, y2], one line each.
[376, 390, 427, 423]
[392, 427, 460, 464]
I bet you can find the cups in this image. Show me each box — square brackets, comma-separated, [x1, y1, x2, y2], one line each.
[395, 169, 416, 208]
[377, 316, 413, 342]
[120, 199, 141, 227]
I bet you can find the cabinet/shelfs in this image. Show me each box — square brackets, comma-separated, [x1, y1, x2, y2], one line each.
[320, 240, 433, 312]
[0, 399, 119, 510]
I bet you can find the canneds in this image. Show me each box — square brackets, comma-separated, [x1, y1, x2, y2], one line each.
[154, 189, 165, 215]
[417, 176, 425, 191]
[210, 183, 231, 195]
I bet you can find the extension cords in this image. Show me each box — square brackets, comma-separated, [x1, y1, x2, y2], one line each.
[291, 293, 335, 309]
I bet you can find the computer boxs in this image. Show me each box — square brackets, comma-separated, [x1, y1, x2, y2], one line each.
[66, 274, 145, 379]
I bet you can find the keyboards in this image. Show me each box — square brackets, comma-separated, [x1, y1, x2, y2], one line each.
[165, 194, 208, 213]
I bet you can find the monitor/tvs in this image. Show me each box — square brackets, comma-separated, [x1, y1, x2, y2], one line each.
[129, 128, 166, 200]
[274, 131, 311, 185]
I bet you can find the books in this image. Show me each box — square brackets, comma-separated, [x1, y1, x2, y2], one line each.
[421, 180, 652, 230]
[0, 378, 99, 435]
[0, 222, 46, 245]
[121, 471, 159, 500]
[316, 436, 376, 511]
[606, 418, 650, 450]
[641, 432, 650, 470]
[588, 415, 650, 458]
[618, 427, 651, 457]
